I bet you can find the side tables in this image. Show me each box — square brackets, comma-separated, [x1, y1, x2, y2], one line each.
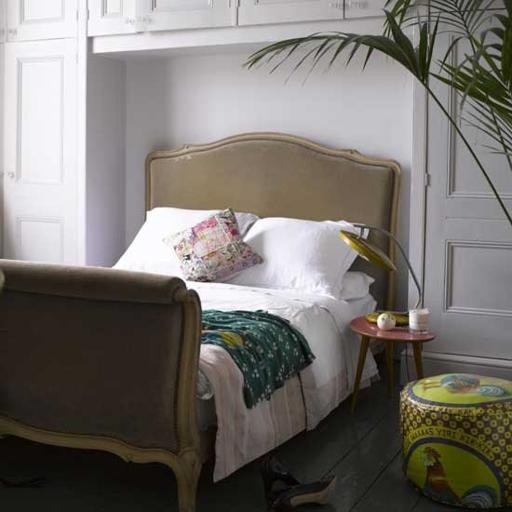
[349, 316, 437, 412]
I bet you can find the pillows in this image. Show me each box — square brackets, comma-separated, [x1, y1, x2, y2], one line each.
[112, 205, 376, 303]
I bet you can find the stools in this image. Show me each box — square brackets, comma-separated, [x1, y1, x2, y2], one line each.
[400, 371, 511, 511]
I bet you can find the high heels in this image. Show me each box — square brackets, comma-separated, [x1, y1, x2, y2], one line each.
[260, 456, 299, 499]
[277, 478, 337, 510]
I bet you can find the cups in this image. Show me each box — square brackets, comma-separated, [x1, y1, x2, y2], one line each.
[408, 309, 430, 336]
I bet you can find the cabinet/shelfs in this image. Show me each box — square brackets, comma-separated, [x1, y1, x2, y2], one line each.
[1, 1, 125, 266]
[398, 3, 512, 385]
[90, 0, 409, 58]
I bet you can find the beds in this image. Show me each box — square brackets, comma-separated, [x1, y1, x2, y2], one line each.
[0, 133, 403, 512]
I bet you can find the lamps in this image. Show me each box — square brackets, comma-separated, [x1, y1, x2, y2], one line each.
[337, 223, 422, 323]
